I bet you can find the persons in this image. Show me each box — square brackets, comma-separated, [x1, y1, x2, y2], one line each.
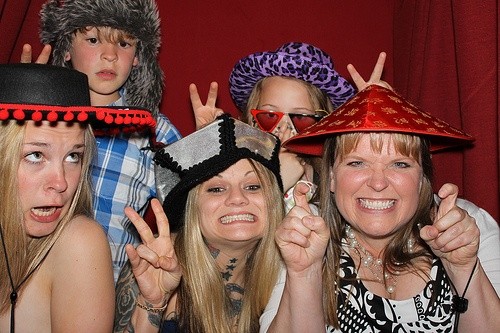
[258, 84, 500, 333]
[112, 112, 285, 333]
[0, 62, 156, 333]
[20, 1, 225, 287]
[227, 42, 394, 213]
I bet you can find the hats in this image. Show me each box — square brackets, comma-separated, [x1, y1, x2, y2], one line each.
[282, 85, 478, 155]
[154, 115, 284, 234]
[0, 63, 157, 136]
[228, 42, 358, 112]
[39, 0, 163, 114]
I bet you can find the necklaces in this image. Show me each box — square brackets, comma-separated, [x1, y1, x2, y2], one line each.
[343, 222, 421, 300]
[205, 243, 246, 282]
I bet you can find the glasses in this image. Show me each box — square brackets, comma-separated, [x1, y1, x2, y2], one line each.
[249, 109, 324, 134]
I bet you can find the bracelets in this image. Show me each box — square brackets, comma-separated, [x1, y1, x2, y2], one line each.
[134, 301, 168, 312]
[446, 258, 478, 333]
